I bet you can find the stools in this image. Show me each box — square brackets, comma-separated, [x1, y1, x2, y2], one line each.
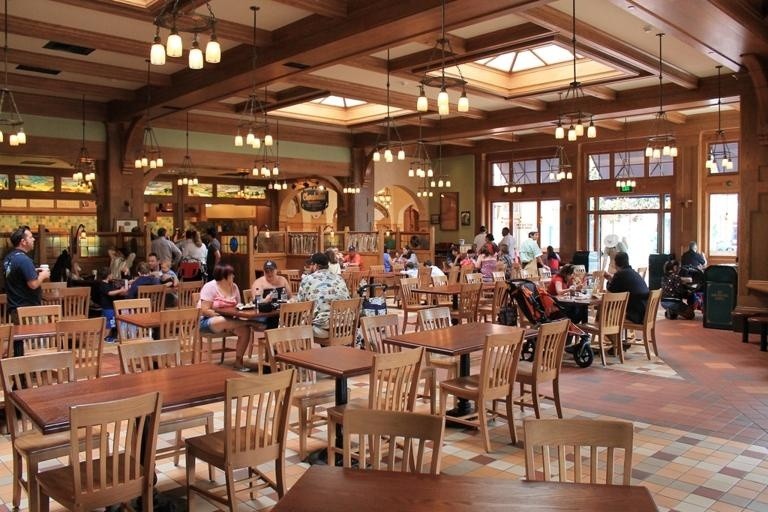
[730, 306, 766, 341]
[747, 314, 765, 349]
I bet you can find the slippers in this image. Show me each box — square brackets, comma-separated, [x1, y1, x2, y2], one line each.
[231, 361, 252, 373]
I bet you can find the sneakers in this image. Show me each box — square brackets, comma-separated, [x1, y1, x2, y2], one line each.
[103, 336, 119, 344]
[664, 307, 677, 319]
[606, 339, 632, 357]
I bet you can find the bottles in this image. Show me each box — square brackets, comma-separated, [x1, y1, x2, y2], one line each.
[253, 286, 289, 304]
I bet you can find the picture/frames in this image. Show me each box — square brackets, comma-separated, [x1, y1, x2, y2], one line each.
[430, 192, 473, 231]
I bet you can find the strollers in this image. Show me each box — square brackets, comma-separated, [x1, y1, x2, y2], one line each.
[350, 281, 391, 347]
[680, 266, 706, 316]
[500, 276, 596, 367]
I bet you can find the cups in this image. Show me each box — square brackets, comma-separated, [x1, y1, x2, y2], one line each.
[561, 275, 601, 300]
[90, 269, 99, 282]
[459, 238, 465, 245]
[39, 264, 50, 281]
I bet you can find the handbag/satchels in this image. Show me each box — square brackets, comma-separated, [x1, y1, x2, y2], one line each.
[497, 302, 518, 327]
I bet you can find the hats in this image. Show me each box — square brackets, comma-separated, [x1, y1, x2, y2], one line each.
[305, 252, 330, 267]
[262, 260, 277, 271]
[348, 245, 355, 251]
[601, 233, 618, 249]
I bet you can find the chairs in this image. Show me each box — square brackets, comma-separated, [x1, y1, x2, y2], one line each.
[524, 419, 634, 486]
[0, 261, 427, 511]
[493, 320, 571, 444]
[361, 314, 436, 416]
[342, 408, 447, 476]
[442, 330, 526, 453]
[418, 307, 483, 409]
[417, 262, 664, 366]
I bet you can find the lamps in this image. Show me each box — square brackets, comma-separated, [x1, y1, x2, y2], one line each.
[71, 91, 201, 187]
[413, 0, 472, 117]
[551, 2, 599, 142]
[406, 107, 453, 198]
[546, 142, 572, 180]
[264, 176, 287, 191]
[702, 60, 738, 171]
[0, 1, 29, 148]
[501, 130, 524, 193]
[611, 115, 636, 187]
[246, 137, 282, 178]
[234, 4, 275, 148]
[371, 47, 408, 163]
[146, 0, 223, 72]
[644, 31, 678, 159]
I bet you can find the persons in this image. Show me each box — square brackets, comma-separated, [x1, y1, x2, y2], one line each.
[2, 223, 51, 391]
[604, 252, 649, 355]
[604, 234, 628, 277]
[196, 262, 267, 371]
[296, 247, 353, 339]
[446, 226, 561, 282]
[384, 245, 420, 273]
[250, 260, 292, 329]
[546, 263, 590, 347]
[423, 259, 447, 287]
[661, 241, 706, 321]
[343, 246, 360, 267]
[296, 251, 353, 336]
[400, 262, 419, 278]
[52, 222, 222, 345]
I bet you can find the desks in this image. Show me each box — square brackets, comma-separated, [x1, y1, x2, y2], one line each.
[744, 276, 766, 300]
[272, 464, 659, 511]
[383, 322, 541, 430]
